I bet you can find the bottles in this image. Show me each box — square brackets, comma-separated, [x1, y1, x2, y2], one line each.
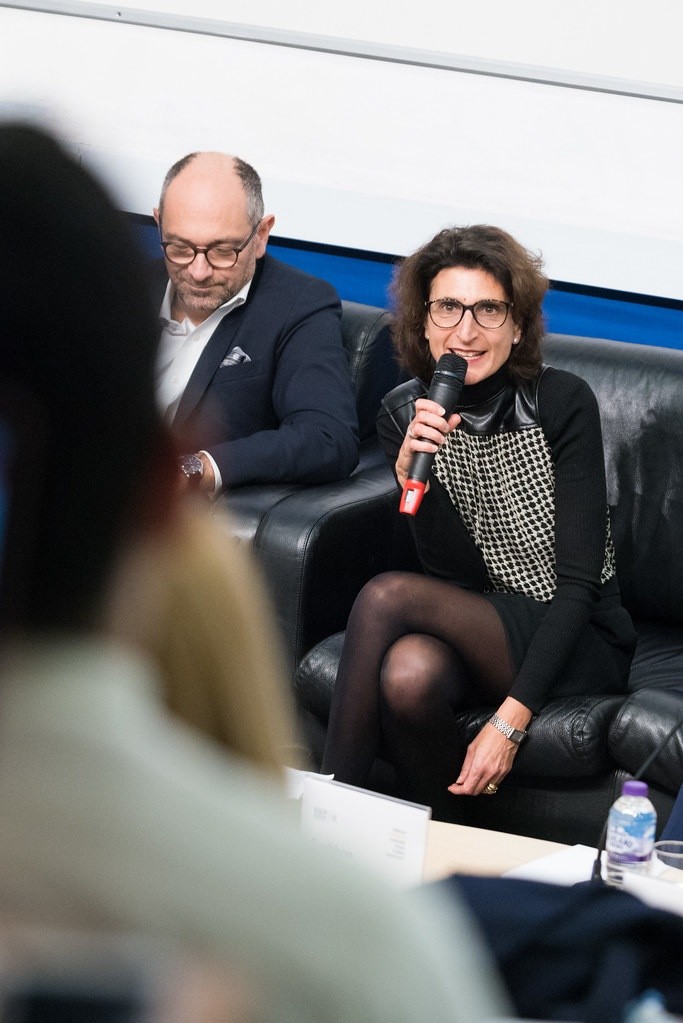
[605, 779, 655, 897]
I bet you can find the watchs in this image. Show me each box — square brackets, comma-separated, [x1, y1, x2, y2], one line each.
[176, 455, 204, 490]
[489, 713, 527, 744]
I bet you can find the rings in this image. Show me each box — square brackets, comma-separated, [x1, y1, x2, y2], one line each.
[408, 430, 418, 439]
[485, 783, 498, 796]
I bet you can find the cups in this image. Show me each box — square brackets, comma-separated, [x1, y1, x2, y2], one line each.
[654, 840, 683, 914]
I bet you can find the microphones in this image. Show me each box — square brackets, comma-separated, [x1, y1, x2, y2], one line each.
[398, 354, 468, 517]
[575, 718, 683, 889]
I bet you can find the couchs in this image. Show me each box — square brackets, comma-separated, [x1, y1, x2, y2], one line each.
[212, 297, 401, 560]
[248, 329, 682, 848]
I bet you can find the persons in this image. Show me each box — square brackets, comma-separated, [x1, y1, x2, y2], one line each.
[138, 151, 360, 501]
[320, 226, 636, 794]
[0, 113, 518, 1021]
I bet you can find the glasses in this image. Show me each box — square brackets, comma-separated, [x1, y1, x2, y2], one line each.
[158, 221, 257, 270]
[425, 298, 514, 330]
